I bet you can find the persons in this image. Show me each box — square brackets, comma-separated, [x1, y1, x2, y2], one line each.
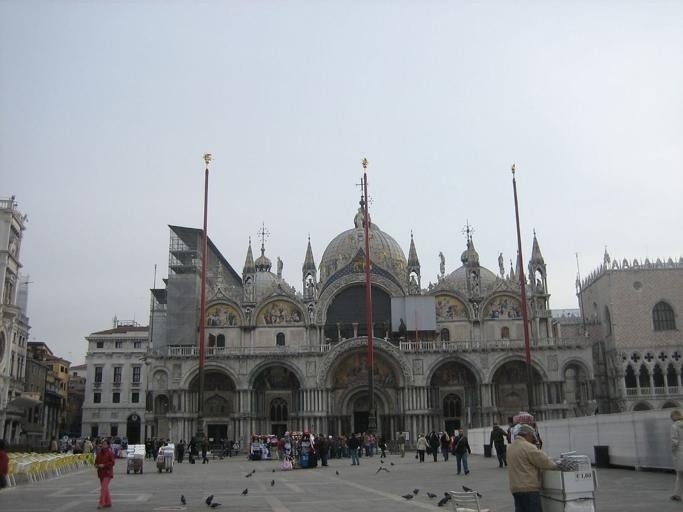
[486, 302, 517, 319]
[0, 440, 10, 493]
[416, 431, 450, 463]
[264, 308, 301, 325]
[176, 433, 209, 465]
[50, 434, 128, 454]
[94, 440, 115, 510]
[436, 300, 458, 320]
[398, 430, 406, 457]
[453, 428, 471, 475]
[145, 437, 166, 460]
[504, 424, 558, 511]
[270, 430, 388, 465]
[224, 437, 240, 457]
[667, 409, 683, 501]
[206, 307, 236, 327]
[490, 415, 543, 468]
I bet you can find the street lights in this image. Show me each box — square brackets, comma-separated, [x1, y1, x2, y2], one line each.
[196, 154, 213, 437]
[361, 158, 377, 434]
[511, 162, 538, 420]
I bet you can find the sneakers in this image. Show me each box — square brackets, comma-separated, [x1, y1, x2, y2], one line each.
[670, 495, 680, 500]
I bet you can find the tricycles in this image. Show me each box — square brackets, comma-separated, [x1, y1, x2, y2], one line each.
[127, 459, 144, 474]
[156, 455, 175, 472]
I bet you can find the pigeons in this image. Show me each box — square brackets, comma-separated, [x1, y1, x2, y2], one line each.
[208, 502, 221, 508]
[391, 462, 395, 466]
[401, 488, 452, 507]
[272, 468, 275, 472]
[336, 470, 339, 475]
[470, 490, 483, 498]
[462, 485, 471, 492]
[380, 459, 384, 464]
[240, 488, 248, 496]
[244, 468, 255, 477]
[205, 495, 213, 504]
[271, 479, 274, 486]
[181, 494, 186, 505]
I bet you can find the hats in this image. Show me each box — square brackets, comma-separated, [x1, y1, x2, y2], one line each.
[519, 424, 538, 441]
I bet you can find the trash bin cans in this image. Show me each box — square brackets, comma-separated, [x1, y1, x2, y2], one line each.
[484, 444, 492, 458]
[593, 445, 610, 468]
